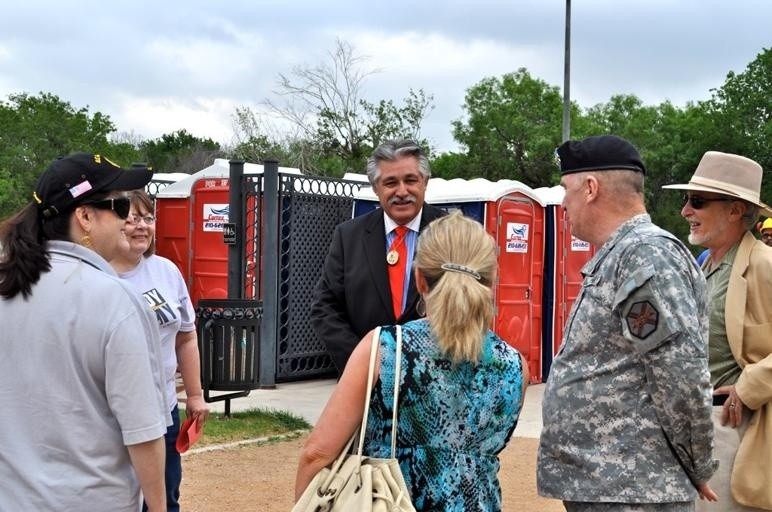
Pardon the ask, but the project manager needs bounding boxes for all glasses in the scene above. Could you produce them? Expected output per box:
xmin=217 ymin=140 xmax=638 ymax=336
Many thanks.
xmin=678 ymin=193 xmax=734 ymax=209
xmin=125 ymin=214 xmax=158 ymax=227
xmin=79 ymin=195 xmax=130 ymax=219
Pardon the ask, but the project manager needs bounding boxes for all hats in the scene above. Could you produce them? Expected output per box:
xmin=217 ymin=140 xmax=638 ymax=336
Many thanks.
xmin=662 ymin=151 xmax=765 ymax=207
xmin=557 ymin=136 xmax=645 ymax=174
xmin=761 ymin=218 xmax=772 ymax=231
xmin=30 ymin=152 xmax=154 ymax=220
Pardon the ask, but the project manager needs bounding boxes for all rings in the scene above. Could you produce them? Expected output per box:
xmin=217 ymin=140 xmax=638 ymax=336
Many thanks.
xmin=729 ymin=402 xmax=735 ymax=410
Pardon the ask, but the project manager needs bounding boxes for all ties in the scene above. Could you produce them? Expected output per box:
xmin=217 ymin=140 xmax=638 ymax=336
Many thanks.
xmin=388 ymin=225 xmax=406 ymax=320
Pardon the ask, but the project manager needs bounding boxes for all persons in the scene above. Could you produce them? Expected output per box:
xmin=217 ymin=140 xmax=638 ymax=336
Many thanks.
xmin=294 ymin=211 xmax=529 ymax=512
xmin=538 ymin=135 xmax=717 ymax=512
xmin=107 ymin=190 xmax=208 ymax=512
xmin=663 ymin=150 xmax=772 ymax=512
xmin=0 ymin=154 xmax=168 ymax=512
xmin=760 ymin=218 xmax=772 ymax=247
xmin=312 ymin=138 xmax=449 ymax=379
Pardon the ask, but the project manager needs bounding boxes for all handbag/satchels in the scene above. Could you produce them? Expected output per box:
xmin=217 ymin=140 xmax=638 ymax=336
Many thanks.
xmin=290 ymin=324 xmax=415 ymax=512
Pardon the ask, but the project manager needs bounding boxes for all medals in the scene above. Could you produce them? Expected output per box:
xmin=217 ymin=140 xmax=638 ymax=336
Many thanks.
xmin=385 ymin=218 xmax=417 ymax=266
